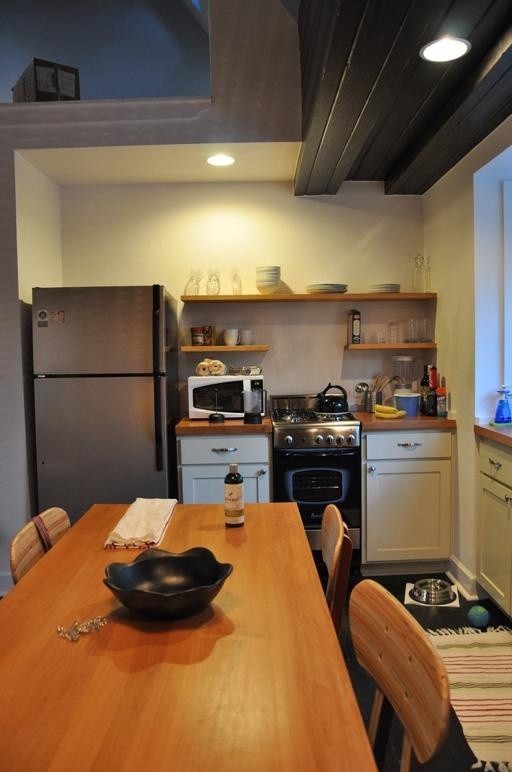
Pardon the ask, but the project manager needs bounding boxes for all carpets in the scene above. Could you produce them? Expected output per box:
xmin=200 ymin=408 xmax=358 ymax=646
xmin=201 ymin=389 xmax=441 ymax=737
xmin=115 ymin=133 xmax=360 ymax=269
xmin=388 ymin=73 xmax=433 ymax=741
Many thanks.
xmin=424 ymin=626 xmax=512 ymax=771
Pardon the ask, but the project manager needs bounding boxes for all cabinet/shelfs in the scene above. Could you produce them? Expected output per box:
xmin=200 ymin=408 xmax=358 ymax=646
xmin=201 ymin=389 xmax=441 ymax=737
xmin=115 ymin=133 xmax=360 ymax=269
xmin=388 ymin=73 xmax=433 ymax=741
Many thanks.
xmin=176 ymin=432 xmax=273 ymax=504
xmin=352 ymin=429 xmax=457 ymax=577
xmin=178 ymin=290 xmax=437 ymax=353
xmin=475 ymin=437 xmax=512 ymax=619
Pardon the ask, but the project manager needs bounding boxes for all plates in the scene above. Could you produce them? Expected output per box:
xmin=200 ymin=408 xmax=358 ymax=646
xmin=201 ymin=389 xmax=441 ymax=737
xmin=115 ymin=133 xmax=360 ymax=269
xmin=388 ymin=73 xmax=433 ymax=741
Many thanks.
xmin=368 ymin=283 xmax=401 ymax=292
xmin=306 ymin=283 xmax=348 ymax=293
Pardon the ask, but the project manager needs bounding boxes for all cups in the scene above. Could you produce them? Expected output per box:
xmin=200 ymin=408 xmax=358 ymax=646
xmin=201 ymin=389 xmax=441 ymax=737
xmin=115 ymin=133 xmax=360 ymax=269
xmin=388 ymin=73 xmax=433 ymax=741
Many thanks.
xmin=373 ymin=331 xmax=386 ymax=343
xmin=224 ymin=328 xmax=241 ymax=345
xmin=361 ymin=331 xmax=373 ymax=343
xmin=387 ymin=321 xmax=399 ymax=344
xmin=240 ymin=329 xmax=254 ymax=346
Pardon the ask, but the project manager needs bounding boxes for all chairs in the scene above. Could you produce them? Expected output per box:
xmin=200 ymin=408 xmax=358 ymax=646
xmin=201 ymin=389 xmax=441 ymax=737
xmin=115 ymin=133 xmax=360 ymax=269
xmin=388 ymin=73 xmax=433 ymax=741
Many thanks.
xmin=349 ymin=578 xmax=450 ymax=771
xmin=319 ymin=504 xmax=351 ymax=642
xmin=11 ymin=507 xmax=71 ymax=587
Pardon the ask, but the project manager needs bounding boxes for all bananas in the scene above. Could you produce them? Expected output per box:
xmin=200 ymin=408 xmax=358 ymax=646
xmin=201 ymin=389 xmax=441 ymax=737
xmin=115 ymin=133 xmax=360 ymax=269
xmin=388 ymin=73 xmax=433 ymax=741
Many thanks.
xmin=375 ymin=404 xmax=408 ymax=419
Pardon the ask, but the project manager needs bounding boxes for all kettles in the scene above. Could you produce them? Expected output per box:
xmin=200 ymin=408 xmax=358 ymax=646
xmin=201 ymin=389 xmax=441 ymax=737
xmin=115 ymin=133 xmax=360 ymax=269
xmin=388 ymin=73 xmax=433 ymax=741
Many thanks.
xmin=313 ymin=382 xmax=349 ymax=412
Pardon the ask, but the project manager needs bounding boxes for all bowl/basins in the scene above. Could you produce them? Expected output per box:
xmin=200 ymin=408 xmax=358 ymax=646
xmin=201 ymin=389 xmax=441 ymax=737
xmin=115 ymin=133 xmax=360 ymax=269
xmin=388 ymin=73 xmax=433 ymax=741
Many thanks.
xmin=255 ymin=265 xmax=282 ymax=295
xmin=101 ymin=546 xmax=234 ymax=619
xmin=409 ymin=578 xmax=457 ymax=605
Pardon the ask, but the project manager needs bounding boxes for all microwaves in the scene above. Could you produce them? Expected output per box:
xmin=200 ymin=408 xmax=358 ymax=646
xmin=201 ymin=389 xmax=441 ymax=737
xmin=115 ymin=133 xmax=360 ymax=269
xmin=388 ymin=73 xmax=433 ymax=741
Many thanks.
xmin=187 ymin=375 xmax=268 ymax=420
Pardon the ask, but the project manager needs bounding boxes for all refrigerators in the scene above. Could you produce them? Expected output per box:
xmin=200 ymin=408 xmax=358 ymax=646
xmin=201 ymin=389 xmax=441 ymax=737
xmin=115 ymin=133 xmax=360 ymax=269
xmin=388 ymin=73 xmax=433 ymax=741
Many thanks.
xmin=31 ymin=284 xmax=181 ymax=514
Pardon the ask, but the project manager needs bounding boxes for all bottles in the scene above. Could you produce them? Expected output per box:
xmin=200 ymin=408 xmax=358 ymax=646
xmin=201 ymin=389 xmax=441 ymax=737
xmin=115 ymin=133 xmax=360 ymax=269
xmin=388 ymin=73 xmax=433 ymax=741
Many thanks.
xmin=414 ymin=255 xmax=427 ymax=293
xmin=421 ymin=364 xmax=429 ymax=412
xmin=348 ymin=305 xmax=362 ymax=344
xmin=190 ymin=326 xmax=203 ymax=346
xmin=223 ymin=464 xmax=245 ymax=529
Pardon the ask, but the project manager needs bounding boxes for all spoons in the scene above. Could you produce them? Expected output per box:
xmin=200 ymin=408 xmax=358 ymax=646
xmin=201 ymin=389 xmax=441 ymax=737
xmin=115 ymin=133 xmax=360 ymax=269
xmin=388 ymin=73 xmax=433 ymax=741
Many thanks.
xmin=355 ymin=382 xmax=370 ymax=392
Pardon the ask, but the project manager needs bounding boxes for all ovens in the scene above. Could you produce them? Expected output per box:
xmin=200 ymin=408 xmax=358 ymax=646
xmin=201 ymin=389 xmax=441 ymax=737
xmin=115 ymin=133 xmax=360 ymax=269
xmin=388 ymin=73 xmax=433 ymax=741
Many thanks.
xmin=272 ymin=446 xmax=362 ymax=530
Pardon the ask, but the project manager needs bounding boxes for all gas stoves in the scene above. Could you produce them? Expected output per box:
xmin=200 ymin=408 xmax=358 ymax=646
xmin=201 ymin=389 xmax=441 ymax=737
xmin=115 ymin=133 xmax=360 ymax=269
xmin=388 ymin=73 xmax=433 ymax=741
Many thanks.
xmin=268 ymin=410 xmax=361 ymax=449
